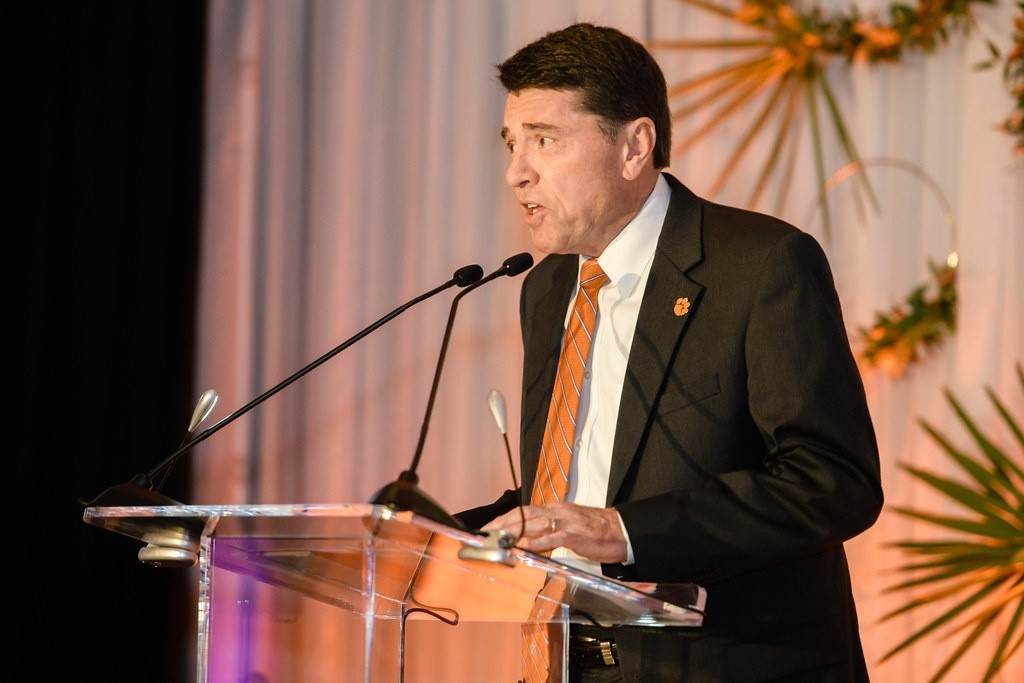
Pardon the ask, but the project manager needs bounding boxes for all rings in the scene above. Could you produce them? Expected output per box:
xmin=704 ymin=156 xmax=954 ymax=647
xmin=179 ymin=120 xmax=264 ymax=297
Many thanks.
xmin=550 ymin=517 xmax=557 ymax=532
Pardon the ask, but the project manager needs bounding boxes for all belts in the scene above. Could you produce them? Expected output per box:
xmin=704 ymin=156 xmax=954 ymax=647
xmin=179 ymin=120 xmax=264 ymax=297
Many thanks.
xmin=570 ymin=635 xmax=621 ymax=667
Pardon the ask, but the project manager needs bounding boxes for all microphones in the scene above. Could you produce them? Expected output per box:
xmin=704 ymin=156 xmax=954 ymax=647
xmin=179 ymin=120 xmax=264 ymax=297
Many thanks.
xmin=367 ymin=253 xmax=534 ymax=535
xmin=90 ymin=264 xmax=484 ymax=507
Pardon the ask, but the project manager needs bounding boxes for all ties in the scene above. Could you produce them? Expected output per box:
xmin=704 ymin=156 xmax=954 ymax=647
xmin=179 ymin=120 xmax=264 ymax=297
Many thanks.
xmin=521 ymin=573 xmax=570 ymax=683
xmin=529 ymin=258 xmax=607 ymax=559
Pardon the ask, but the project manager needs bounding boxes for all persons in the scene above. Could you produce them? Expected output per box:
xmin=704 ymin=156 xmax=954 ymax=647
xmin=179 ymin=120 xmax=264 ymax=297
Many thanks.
xmin=447 ymin=22 xmax=886 ymax=683
